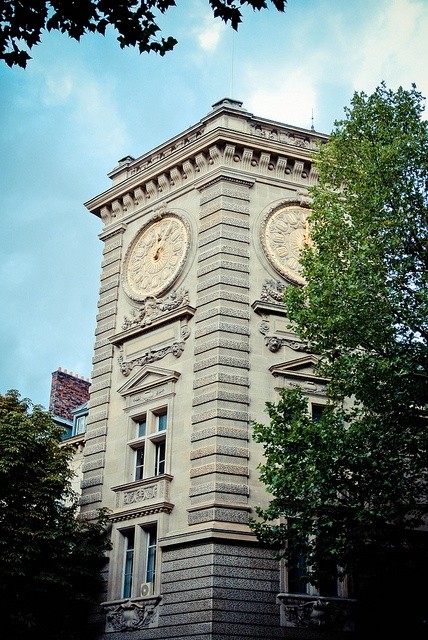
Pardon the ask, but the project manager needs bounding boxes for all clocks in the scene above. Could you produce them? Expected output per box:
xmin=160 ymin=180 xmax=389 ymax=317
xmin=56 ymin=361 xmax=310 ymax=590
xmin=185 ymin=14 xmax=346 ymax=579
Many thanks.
xmin=120 ymin=209 xmax=198 ymax=307
xmin=252 ymin=196 xmax=350 ymax=289
